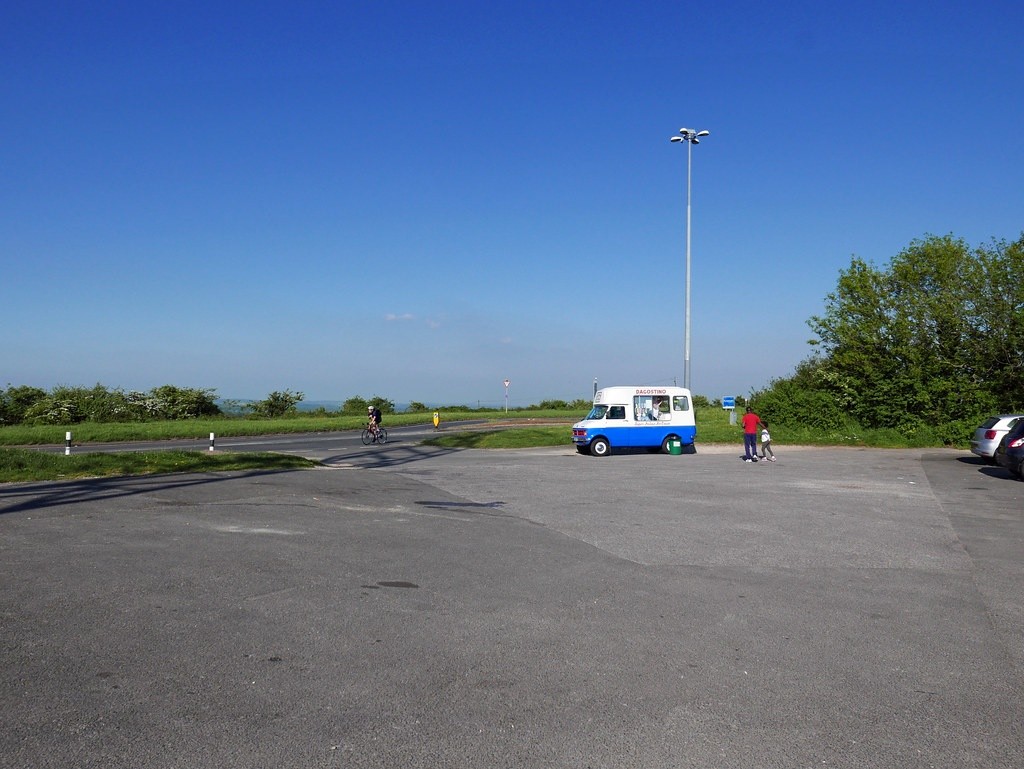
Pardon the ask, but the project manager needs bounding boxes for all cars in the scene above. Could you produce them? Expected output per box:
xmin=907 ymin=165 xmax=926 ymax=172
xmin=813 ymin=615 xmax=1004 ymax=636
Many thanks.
xmin=968 ymin=416 xmax=1024 ymax=479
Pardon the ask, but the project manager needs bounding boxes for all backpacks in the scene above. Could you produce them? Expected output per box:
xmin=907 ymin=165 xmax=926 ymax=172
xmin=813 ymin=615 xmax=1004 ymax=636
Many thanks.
xmin=375 ymin=408 xmax=382 ymax=418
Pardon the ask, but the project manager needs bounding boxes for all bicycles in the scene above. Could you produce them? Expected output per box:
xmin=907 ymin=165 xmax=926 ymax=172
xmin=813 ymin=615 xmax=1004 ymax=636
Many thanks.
xmin=361 ymin=423 xmax=387 ymax=445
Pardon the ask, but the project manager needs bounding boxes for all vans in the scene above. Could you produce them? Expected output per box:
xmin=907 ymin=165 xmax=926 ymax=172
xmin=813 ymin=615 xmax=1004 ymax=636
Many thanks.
xmin=570 ymin=386 xmax=696 ymax=457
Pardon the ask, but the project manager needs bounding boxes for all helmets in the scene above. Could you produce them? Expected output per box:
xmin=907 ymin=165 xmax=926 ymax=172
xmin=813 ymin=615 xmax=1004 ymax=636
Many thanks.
xmin=368 ymin=406 xmax=374 ymax=409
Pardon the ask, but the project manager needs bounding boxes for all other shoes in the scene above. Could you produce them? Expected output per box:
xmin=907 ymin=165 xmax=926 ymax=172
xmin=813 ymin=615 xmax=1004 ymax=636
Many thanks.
xmin=375 ymin=430 xmax=380 ymax=435
xmin=372 ymin=438 xmax=376 ymax=443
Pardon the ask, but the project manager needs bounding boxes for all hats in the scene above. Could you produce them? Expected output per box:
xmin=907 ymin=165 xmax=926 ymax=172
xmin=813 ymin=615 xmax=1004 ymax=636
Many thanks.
xmin=746 ymin=406 xmax=754 ymax=411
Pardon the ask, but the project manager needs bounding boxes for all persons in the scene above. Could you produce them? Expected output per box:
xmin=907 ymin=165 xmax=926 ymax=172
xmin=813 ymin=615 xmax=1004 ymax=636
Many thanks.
xmin=760 ymin=421 xmax=776 ymax=462
xmin=367 ymin=406 xmax=381 ymax=434
xmin=742 ymin=407 xmax=768 ymax=462
xmin=653 ymin=400 xmax=662 ymax=420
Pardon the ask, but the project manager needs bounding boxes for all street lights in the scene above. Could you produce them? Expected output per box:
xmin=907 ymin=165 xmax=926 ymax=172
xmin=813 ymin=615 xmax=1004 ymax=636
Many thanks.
xmin=671 ymin=128 xmax=709 ymax=411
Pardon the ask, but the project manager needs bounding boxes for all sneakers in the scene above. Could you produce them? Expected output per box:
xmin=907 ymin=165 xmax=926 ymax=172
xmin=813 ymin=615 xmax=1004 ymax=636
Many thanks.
xmin=762 ymin=457 xmax=767 ymax=461
xmin=745 ymin=459 xmax=752 ymax=463
xmin=770 ymin=456 xmax=776 ymax=461
xmin=753 ymin=455 xmax=758 ymax=461
